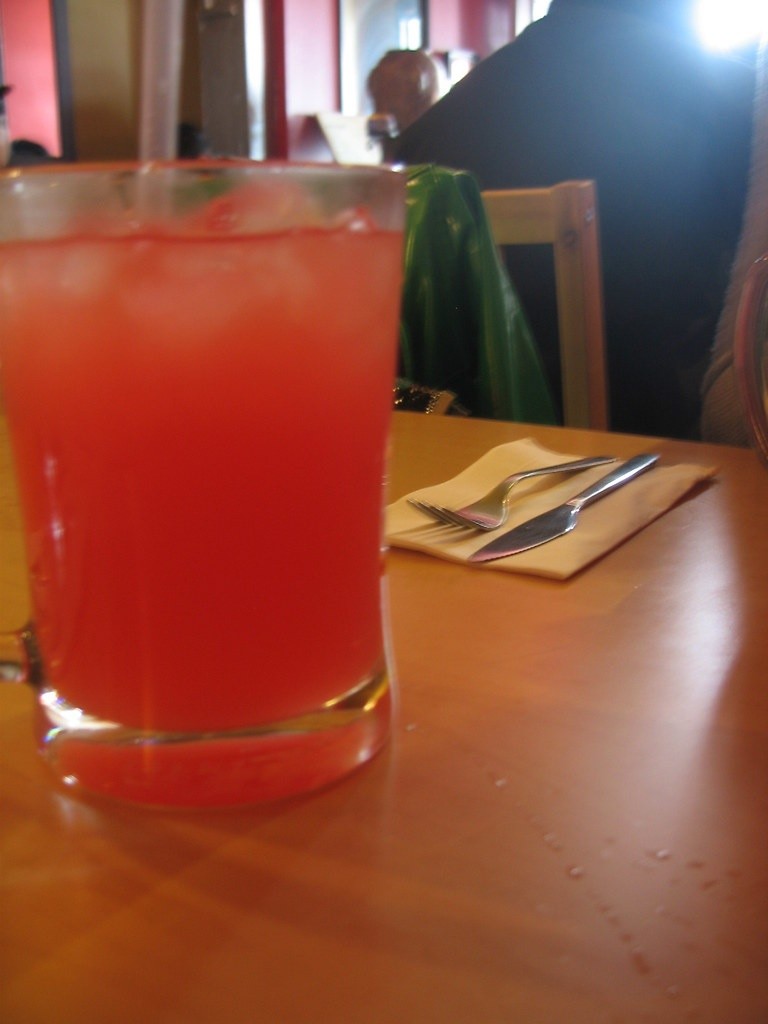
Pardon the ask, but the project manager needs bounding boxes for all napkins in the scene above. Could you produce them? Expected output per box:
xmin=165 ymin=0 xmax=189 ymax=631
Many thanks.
xmin=385 ymin=434 xmax=716 ymax=586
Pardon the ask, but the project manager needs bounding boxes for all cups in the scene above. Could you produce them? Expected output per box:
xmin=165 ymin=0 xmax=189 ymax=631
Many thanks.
xmin=0 ymin=163 xmax=409 ymax=811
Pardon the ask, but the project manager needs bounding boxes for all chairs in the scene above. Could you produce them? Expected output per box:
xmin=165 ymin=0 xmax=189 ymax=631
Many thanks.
xmin=392 ymin=179 xmax=611 ymax=434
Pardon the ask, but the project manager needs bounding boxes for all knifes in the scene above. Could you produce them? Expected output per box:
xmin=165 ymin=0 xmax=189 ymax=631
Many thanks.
xmin=467 ymin=452 xmax=662 ymax=564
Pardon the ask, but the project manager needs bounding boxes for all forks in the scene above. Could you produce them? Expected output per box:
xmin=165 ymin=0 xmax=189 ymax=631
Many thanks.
xmin=408 ymin=456 xmax=614 ymax=532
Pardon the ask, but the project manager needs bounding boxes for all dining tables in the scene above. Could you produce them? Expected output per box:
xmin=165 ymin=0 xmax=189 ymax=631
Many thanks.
xmin=0 ymin=407 xmax=768 ymax=1024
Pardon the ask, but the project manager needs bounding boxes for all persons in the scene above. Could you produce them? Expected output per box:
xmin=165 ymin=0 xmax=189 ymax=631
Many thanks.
xmin=386 ymin=0 xmax=768 ymax=445
